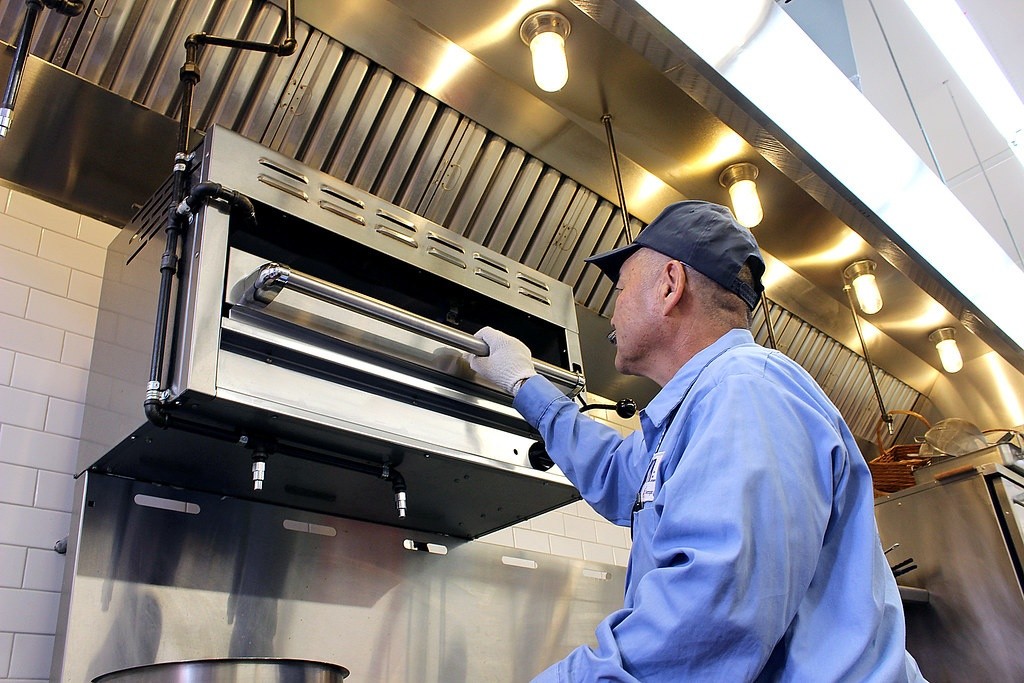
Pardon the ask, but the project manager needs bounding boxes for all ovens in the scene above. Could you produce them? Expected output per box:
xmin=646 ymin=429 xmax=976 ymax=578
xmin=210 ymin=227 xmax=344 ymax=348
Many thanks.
xmin=72 ymin=121 xmax=590 ymax=542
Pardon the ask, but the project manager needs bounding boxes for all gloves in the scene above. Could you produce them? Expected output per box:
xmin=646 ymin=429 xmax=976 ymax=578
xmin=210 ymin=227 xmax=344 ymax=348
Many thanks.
xmin=467 ymin=326 xmax=538 ymax=398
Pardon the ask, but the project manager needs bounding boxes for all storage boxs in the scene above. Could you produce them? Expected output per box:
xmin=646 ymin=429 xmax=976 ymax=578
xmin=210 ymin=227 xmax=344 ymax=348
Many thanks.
xmin=911 ymin=441 xmax=1015 ymax=485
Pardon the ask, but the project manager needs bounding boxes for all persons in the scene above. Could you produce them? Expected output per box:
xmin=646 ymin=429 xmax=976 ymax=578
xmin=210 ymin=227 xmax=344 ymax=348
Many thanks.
xmin=469 ymin=199 xmax=930 ymax=683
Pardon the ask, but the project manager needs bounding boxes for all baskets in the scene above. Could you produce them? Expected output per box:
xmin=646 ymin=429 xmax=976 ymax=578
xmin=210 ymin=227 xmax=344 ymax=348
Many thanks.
xmin=869 ymin=411 xmax=932 ymax=493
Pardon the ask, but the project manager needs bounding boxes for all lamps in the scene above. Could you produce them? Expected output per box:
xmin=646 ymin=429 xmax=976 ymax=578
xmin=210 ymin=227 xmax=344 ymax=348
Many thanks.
xmin=519 ymin=10 xmax=572 ymax=94
xmin=928 ymin=326 xmax=964 ymax=374
xmin=718 ymin=162 xmax=766 ymax=229
xmin=844 ymin=259 xmax=883 ymax=316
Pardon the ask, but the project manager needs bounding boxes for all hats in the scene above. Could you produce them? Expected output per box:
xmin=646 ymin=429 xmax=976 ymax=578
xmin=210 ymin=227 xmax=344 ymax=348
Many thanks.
xmin=583 ymin=200 xmax=765 ymax=312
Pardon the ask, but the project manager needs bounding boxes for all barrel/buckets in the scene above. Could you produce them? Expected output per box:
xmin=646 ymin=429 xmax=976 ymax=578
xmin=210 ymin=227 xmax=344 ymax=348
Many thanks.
xmin=90 ymin=656 xmax=350 ymax=682
